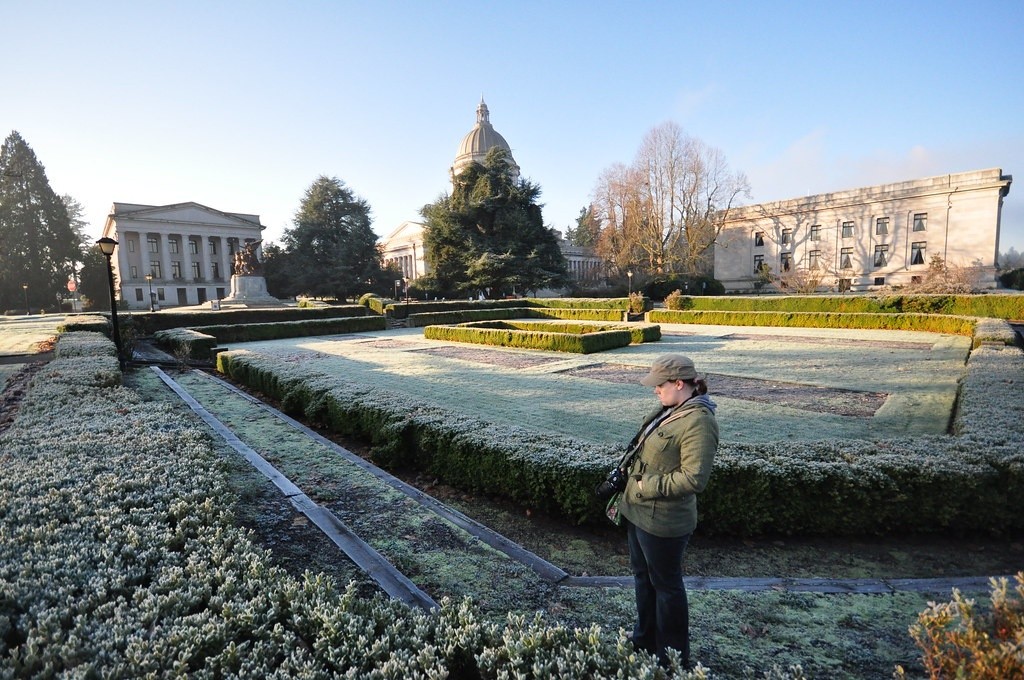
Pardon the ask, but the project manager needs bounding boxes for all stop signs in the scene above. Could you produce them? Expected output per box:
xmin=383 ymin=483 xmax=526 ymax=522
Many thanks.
xmin=67 ymin=281 xmax=77 ymax=292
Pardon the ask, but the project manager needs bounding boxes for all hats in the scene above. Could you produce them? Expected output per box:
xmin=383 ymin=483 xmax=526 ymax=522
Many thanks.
xmin=639 ymin=354 xmax=697 ymax=387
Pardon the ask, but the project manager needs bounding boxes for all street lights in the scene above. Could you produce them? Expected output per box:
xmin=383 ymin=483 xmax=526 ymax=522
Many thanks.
xmin=402 ymin=276 xmax=410 ymax=304
xmin=95 ymin=237 xmax=125 ymax=373
xmin=22 ymin=283 xmax=30 ymax=315
xmin=627 ymin=270 xmax=634 ymax=298
xmin=145 ymin=274 xmax=155 ymax=312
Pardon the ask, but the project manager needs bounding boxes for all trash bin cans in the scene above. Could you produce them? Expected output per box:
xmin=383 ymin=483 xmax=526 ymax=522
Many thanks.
xmin=211 ymin=300 xmax=221 ymax=311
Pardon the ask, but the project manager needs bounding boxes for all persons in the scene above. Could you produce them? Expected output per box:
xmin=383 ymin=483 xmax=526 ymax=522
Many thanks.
xmin=234 ymin=242 xmax=254 ymax=276
xmin=608 ymin=354 xmax=719 ymax=668
